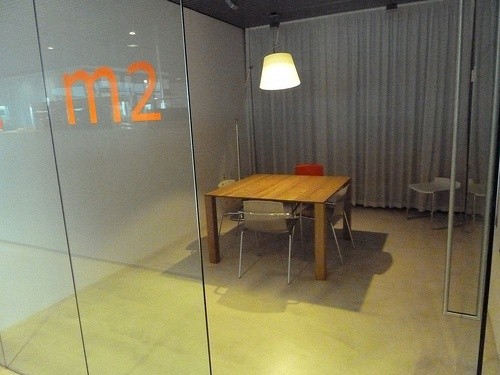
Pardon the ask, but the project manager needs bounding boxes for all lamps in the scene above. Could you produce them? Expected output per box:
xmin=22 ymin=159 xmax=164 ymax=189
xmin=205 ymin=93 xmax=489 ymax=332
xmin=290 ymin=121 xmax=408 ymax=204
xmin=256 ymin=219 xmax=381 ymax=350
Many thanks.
xmin=258 ymin=14 xmax=302 ymax=93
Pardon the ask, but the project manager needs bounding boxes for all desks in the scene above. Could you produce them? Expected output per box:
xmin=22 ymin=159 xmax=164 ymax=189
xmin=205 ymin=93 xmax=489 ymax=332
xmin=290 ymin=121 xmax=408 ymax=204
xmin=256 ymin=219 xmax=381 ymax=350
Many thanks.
xmin=202 ymin=170 xmax=354 ymax=281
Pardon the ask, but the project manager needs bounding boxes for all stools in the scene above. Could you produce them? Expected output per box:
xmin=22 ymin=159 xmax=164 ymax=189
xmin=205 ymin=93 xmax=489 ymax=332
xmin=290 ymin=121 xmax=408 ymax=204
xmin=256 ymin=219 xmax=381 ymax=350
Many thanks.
xmin=467 ymin=179 xmax=487 ymax=231
xmin=403 ymin=177 xmax=462 ymax=228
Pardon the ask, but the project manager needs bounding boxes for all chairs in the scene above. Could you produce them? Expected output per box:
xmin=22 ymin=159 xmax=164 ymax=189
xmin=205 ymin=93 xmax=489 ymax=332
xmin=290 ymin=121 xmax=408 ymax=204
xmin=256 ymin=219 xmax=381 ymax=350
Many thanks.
xmin=296 ymin=180 xmax=354 ymax=264
xmin=236 ymin=199 xmax=299 ymax=285
xmin=216 ymin=179 xmax=264 ymax=249
xmin=293 ymin=162 xmax=325 ymax=176
